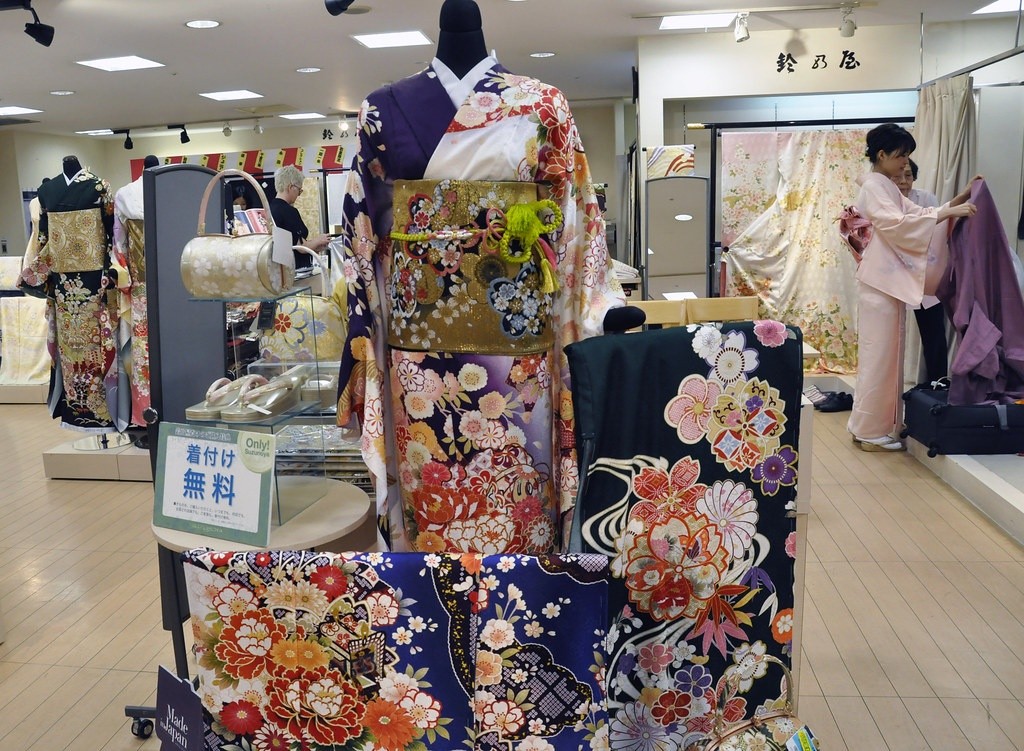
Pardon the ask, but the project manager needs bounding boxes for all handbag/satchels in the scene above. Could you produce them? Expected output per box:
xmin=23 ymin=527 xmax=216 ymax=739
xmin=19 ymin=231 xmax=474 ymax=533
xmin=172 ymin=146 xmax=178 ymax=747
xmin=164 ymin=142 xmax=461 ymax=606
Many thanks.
xmin=180 ymin=169 xmax=295 ymax=298
xmin=259 ymin=245 xmax=347 ymax=362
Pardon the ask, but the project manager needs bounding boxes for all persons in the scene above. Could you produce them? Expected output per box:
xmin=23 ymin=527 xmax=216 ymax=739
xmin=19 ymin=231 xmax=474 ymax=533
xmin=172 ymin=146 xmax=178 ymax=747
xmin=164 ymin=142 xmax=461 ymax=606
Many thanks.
xmin=224 ymin=186 xmax=253 ymax=236
xmin=16 ymin=155 xmax=159 ymax=435
xmin=337 ymin=0 xmax=626 ymax=555
xmin=846 ymin=124 xmax=984 ymax=453
xmin=270 ymin=167 xmax=332 ymax=268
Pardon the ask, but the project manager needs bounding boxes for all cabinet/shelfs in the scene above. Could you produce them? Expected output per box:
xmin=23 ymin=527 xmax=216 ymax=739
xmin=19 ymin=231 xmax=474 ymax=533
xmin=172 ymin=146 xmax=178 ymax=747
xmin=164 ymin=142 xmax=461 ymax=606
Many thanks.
xmin=182 ymin=282 xmax=327 ymax=527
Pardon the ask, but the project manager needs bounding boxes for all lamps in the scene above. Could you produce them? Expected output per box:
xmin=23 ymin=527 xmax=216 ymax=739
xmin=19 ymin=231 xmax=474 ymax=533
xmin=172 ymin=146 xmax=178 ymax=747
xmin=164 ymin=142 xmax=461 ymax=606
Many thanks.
xmin=838 ymin=4 xmax=856 ymax=37
xmin=23 ymin=0 xmax=54 ymax=46
xmin=735 ymin=12 xmax=752 ymax=42
xmin=325 ymin=0 xmax=355 ymax=16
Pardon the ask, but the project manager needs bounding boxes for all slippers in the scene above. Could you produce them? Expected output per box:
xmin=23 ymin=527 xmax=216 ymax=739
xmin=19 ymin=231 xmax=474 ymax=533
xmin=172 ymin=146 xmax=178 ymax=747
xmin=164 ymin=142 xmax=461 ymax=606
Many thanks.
xmin=185 ymin=372 xmax=261 ymax=421
xmin=299 ymin=371 xmax=338 ymax=408
xmin=850 ymin=434 xmax=907 ymax=451
xmin=220 ymin=373 xmax=301 ymax=422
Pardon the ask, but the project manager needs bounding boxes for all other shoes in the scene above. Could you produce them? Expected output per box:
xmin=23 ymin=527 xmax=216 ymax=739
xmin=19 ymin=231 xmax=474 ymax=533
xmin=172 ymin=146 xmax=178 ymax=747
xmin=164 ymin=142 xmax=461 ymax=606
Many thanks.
xmin=813 ymin=391 xmax=846 ymax=408
xmin=820 ymin=394 xmax=854 ymax=412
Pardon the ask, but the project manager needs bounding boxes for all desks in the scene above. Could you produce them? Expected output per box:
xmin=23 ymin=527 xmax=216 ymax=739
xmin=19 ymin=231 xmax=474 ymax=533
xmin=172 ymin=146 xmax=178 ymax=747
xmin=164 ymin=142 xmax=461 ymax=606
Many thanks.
xmin=151 ymin=475 xmax=370 ymax=687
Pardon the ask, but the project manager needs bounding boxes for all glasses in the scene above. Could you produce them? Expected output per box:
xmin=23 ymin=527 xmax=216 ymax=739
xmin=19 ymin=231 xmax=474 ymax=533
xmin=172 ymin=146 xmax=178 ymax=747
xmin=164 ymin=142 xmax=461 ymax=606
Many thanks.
xmin=290 ymin=185 xmax=303 ymax=196
